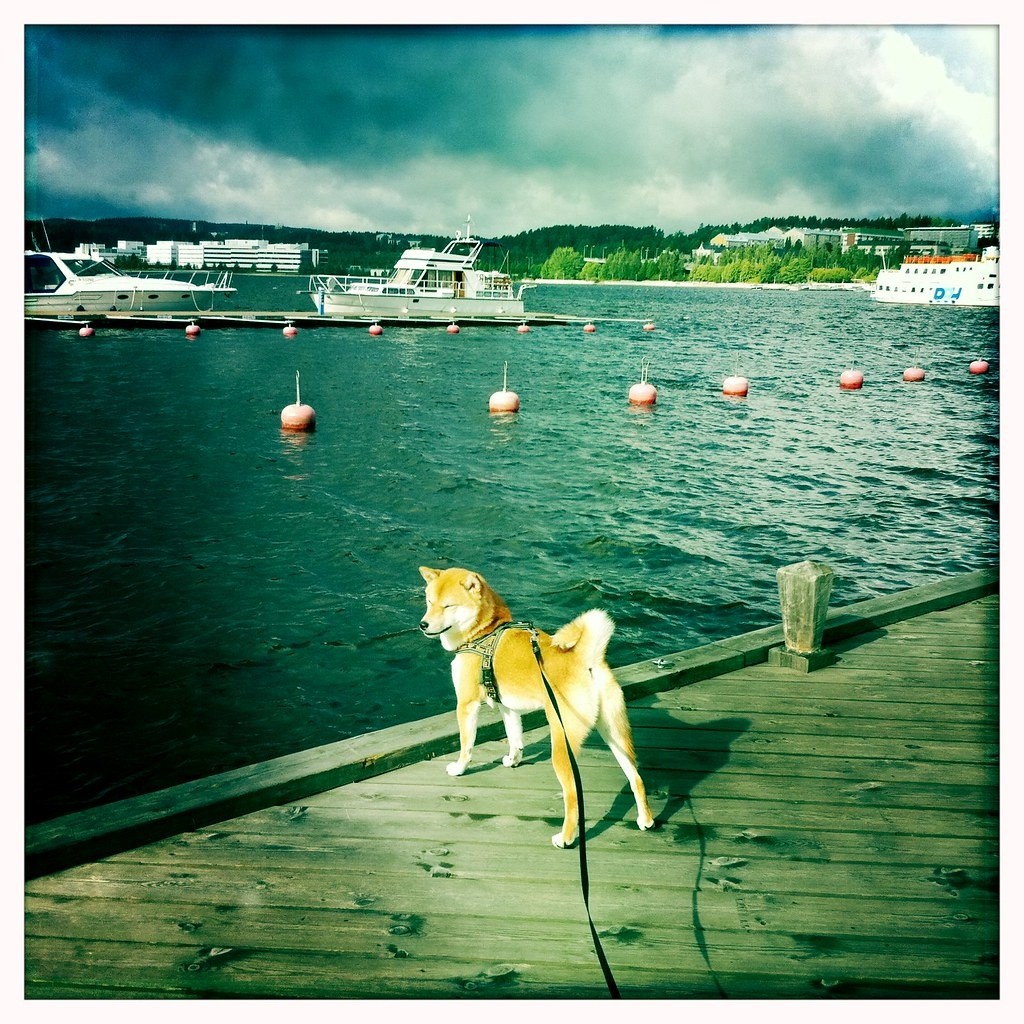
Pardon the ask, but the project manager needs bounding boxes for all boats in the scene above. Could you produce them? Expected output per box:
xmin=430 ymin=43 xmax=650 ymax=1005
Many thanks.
xmin=870 ymin=245 xmax=1000 ymax=306
xmin=24 ymin=250 xmax=238 ymax=311
xmin=296 ymin=214 xmax=537 ymax=312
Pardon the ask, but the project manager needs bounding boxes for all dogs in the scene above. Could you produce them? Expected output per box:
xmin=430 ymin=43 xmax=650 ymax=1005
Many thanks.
xmin=418 ymin=566 xmax=656 ymax=849
